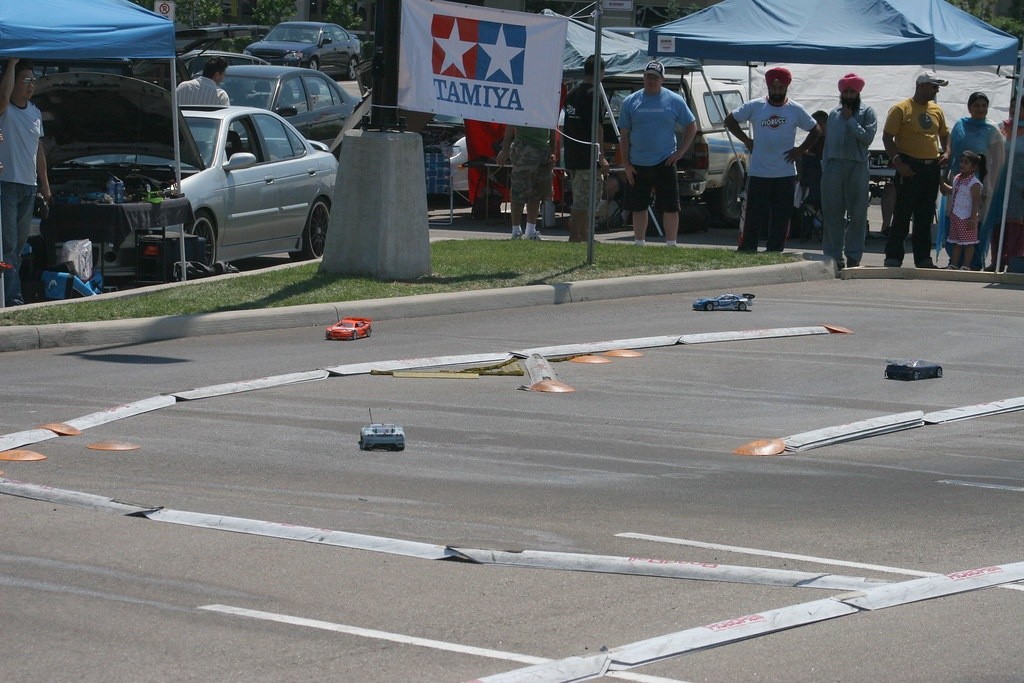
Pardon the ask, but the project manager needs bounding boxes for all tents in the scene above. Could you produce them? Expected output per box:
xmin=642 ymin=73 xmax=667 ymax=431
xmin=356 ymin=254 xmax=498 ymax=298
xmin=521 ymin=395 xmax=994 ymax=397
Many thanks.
xmin=0 ymin=0 xmax=188 ymax=280
xmin=647 ymin=0 xmax=1023 ymax=272
xmin=538 ymin=8 xmax=746 ymax=179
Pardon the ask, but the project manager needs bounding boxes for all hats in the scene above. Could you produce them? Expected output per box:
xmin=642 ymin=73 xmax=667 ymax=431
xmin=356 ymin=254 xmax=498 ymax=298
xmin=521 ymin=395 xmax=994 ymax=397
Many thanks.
xmin=765 ymin=67 xmax=792 ymax=86
xmin=644 ymin=60 xmax=665 ymax=78
xmin=916 ymin=74 xmax=949 ymax=87
xmin=838 ymin=73 xmax=865 ymax=92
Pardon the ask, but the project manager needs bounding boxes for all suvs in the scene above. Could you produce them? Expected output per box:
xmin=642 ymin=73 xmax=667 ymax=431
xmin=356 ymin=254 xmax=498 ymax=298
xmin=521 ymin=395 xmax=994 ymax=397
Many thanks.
xmin=563 ymin=74 xmax=760 ymax=230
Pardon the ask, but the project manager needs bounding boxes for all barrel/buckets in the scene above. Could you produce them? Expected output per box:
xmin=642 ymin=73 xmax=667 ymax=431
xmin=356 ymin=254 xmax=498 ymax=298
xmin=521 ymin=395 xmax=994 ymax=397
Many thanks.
xmin=175 ymin=233 xmax=198 ymax=282
xmin=143 ymin=232 xmax=180 ymax=284
xmin=198 ymin=237 xmax=207 ymax=278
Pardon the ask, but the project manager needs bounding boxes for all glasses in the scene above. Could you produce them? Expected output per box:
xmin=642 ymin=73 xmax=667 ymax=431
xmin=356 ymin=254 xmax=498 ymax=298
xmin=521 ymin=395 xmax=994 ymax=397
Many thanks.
xmin=927 ymin=83 xmax=940 ymax=90
xmin=17 ymin=78 xmax=37 ymax=85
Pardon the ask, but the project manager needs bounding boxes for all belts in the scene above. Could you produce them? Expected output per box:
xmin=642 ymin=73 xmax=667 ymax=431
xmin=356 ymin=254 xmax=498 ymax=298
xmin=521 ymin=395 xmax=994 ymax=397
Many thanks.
xmin=898 ymin=154 xmax=939 ymax=165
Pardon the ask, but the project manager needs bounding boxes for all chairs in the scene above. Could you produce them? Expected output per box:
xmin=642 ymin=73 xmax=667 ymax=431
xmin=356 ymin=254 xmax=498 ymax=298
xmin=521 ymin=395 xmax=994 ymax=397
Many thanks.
xmin=251 ymin=82 xmax=271 ymax=109
xmin=225 ymin=129 xmax=244 ymax=157
xmin=295 ymin=82 xmax=321 ymax=113
xmin=797 ymin=153 xmax=824 ymax=245
xmin=422 ymin=144 xmax=454 ymax=225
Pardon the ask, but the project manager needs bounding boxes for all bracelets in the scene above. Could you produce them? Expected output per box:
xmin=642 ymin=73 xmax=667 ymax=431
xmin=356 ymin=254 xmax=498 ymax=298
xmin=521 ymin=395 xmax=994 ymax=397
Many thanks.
xmin=889 ymin=155 xmax=900 ymax=164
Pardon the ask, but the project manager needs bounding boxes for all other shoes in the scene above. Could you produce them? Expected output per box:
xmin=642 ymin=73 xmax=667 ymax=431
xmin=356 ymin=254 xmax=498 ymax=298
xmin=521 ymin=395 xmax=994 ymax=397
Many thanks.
xmin=970 ymin=264 xmax=981 ymax=271
xmin=837 ymin=263 xmax=845 ymax=274
xmin=916 ymin=264 xmax=938 ymax=268
xmin=984 ymin=263 xmax=1004 ymax=272
xmin=525 ymin=231 xmax=542 ymax=241
xmin=511 ymin=232 xmax=525 ymax=240
xmin=946 ymin=265 xmax=959 ymax=269
xmin=959 ymin=266 xmax=971 ymax=270
xmin=803 ymin=231 xmax=812 ymax=240
xmin=802 ymin=196 xmax=816 ymax=204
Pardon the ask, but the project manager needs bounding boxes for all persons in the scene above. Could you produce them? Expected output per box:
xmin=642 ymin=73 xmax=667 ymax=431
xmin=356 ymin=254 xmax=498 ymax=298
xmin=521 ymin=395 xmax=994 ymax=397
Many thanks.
xmin=984 ymin=92 xmax=1024 ymax=274
xmin=176 ymin=55 xmax=233 ymax=132
xmin=0 ymin=57 xmax=52 ymax=306
xmin=883 ymin=70 xmax=951 ymax=268
xmin=805 ymin=110 xmax=830 ymax=158
xmin=618 ymin=60 xmax=699 ymax=245
xmin=945 ymin=150 xmax=988 ymax=271
xmin=936 ymin=91 xmax=1005 ymax=271
xmin=561 ymin=55 xmax=609 ymax=243
xmin=725 ymin=68 xmax=822 ymax=255
xmin=494 ymin=123 xmax=555 ymax=241
xmin=822 ymin=72 xmax=876 ymax=269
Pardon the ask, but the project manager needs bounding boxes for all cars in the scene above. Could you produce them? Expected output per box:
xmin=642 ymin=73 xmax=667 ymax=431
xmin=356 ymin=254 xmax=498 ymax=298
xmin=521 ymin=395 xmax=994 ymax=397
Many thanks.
xmin=243 ymin=21 xmax=364 ymax=82
xmin=360 ymin=421 xmax=404 ymax=453
xmin=135 ymin=25 xmax=364 ymax=151
xmin=692 ymin=291 xmax=756 ymax=311
xmin=420 ymin=114 xmax=566 ymax=207
xmin=23 ymin=71 xmax=341 ymax=286
xmin=325 ymin=316 xmax=372 ymax=341
xmin=885 ymin=357 xmax=944 ymax=381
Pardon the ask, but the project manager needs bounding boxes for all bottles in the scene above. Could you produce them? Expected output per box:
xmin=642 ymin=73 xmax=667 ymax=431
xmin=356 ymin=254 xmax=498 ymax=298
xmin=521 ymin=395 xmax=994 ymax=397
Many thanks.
xmin=106 ymin=176 xmax=115 ymax=202
xmin=115 ymin=180 xmax=124 ymax=204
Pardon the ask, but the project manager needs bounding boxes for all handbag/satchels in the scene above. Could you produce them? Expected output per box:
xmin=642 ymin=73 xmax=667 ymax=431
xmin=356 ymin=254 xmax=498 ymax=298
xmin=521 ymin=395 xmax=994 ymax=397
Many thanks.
xmin=32 ymin=196 xmax=50 ymax=219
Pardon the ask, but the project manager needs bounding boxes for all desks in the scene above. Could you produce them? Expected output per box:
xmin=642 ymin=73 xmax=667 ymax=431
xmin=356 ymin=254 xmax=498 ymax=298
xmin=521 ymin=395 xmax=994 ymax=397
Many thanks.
xmin=463 ymin=160 xmax=686 ymax=231
xmin=40 ymin=198 xmax=195 ymax=293
xmin=869 ymin=168 xmax=896 ymax=176
